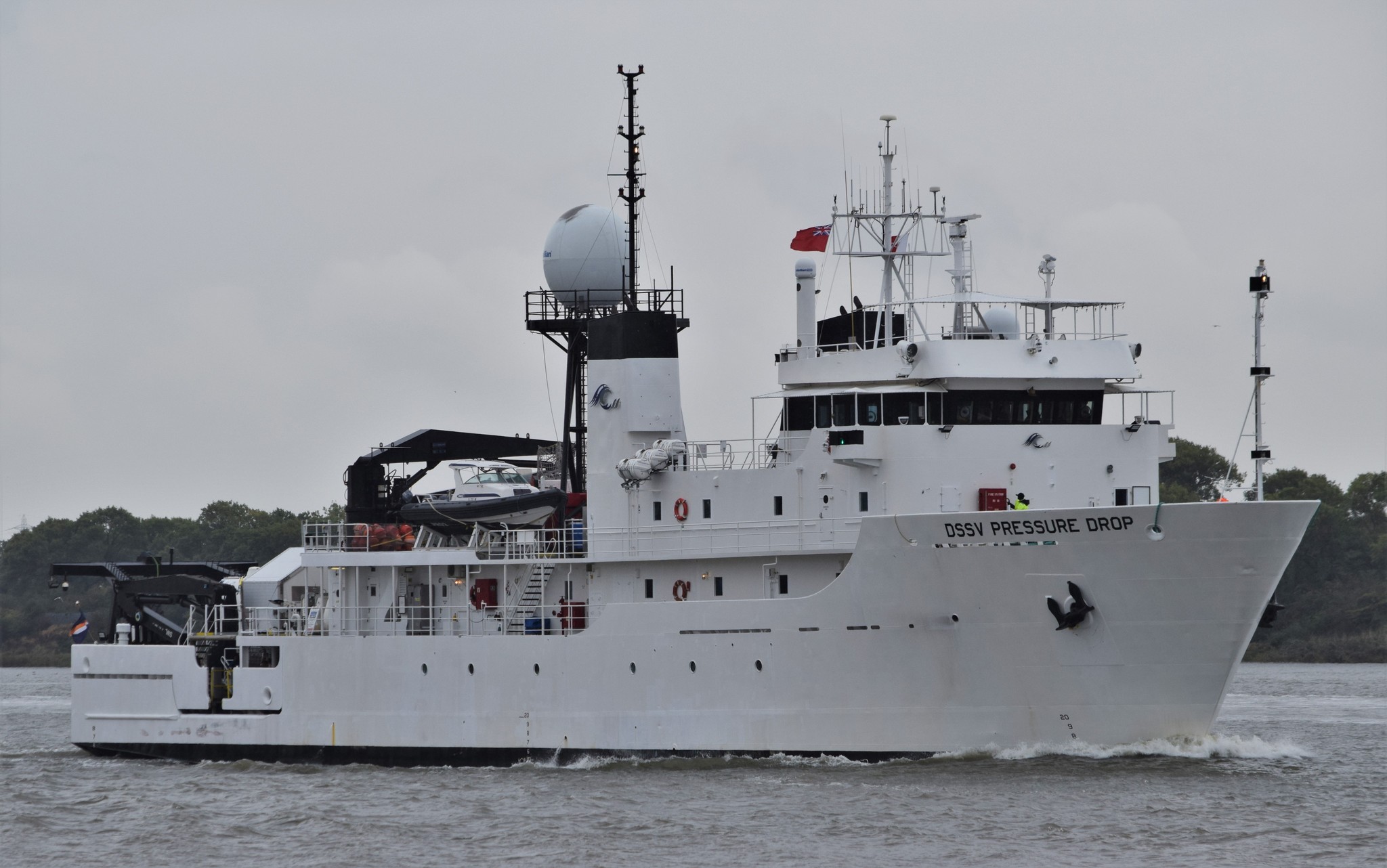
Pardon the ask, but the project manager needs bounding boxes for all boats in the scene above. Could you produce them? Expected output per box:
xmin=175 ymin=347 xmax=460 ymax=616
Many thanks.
xmin=45 ymin=60 xmax=1324 ymax=755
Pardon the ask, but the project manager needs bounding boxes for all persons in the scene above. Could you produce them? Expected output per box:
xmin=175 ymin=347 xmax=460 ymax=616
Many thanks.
xmin=1008 ymin=493 xmax=1029 ymax=510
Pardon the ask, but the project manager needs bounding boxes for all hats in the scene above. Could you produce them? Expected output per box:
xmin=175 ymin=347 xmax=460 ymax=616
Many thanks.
xmin=1016 ymin=493 xmax=1025 ymax=497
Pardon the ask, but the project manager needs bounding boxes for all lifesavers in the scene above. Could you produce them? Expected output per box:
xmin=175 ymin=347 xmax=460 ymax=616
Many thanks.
xmin=674 ymin=498 xmax=688 ymax=521
xmin=673 ymin=581 xmax=688 ymax=602
xmin=470 ymin=585 xmax=476 ymax=606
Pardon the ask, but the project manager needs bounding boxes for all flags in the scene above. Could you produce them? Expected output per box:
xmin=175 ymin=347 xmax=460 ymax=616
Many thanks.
xmin=69 ymin=607 xmax=90 ymax=644
xmin=883 ymin=236 xmax=898 ymax=260
xmin=790 ymin=224 xmax=833 ymax=252
